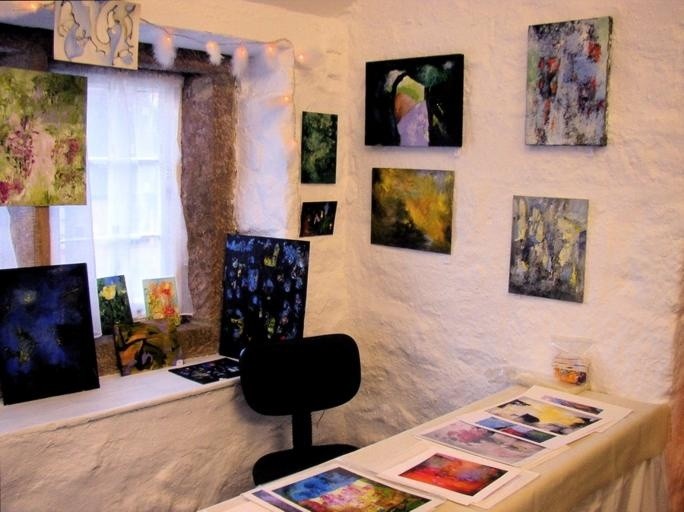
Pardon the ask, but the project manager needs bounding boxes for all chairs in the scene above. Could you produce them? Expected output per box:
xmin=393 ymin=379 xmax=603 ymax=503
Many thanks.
xmin=238 ymin=334 xmax=363 ymax=490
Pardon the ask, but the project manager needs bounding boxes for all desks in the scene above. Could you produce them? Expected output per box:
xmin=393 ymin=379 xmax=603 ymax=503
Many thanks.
xmin=190 ymin=379 xmax=669 ymax=512
